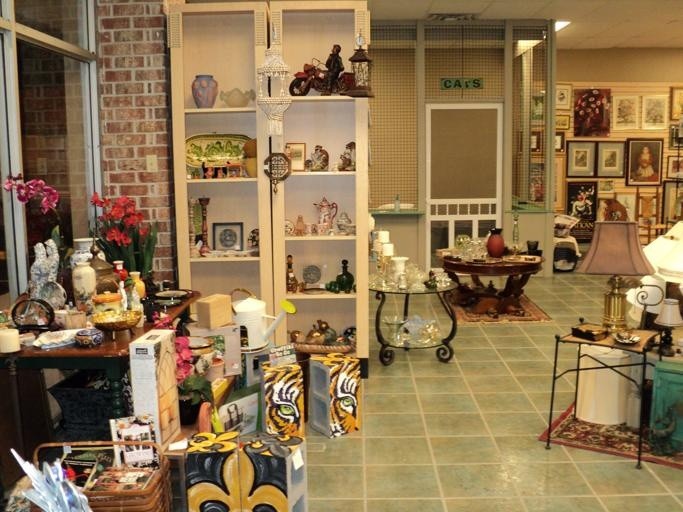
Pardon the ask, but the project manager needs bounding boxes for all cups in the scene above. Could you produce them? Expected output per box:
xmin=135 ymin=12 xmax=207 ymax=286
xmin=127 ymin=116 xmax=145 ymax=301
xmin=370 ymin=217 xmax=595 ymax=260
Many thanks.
xmin=384 ymin=320 xmax=408 ymax=343
xmin=527 ymin=240 xmax=540 ymax=253
xmin=451 ymin=240 xmax=486 ymax=262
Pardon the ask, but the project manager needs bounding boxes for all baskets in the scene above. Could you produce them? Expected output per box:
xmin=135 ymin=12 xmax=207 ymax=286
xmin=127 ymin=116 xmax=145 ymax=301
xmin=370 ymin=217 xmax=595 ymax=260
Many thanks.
xmin=32 ymin=441 xmax=175 ymax=512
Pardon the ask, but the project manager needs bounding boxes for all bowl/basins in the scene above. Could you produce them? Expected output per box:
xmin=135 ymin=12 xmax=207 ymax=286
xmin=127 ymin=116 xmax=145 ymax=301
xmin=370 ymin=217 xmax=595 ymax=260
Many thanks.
xmin=90 ymin=309 xmax=143 ymax=331
xmin=40 ymin=282 xmax=68 ymax=311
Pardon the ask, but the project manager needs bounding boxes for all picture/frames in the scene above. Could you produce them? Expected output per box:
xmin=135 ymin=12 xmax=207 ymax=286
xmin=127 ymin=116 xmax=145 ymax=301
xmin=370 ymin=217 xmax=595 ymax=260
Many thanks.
xmin=283 ymin=141 xmax=307 ymax=173
xmin=524 ymin=82 xmax=683 ymax=243
xmin=211 ymin=222 xmax=244 ymax=252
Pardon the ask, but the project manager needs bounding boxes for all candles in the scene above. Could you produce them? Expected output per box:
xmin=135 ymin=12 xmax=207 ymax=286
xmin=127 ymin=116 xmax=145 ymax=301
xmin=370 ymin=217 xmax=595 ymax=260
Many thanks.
xmin=1 ymin=329 xmax=21 ymax=354
xmin=376 ymin=229 xmax=390 ymax=243
xmin=380 ymin=243 xmax=394 ymax=256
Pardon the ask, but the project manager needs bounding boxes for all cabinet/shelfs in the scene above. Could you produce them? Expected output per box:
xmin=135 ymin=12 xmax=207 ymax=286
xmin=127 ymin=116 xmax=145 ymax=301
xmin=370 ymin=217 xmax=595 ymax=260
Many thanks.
xmin=268 ymin=0 xmax=377 ymax=379
xmin=165 ymin=1 xmax=273 ymax=348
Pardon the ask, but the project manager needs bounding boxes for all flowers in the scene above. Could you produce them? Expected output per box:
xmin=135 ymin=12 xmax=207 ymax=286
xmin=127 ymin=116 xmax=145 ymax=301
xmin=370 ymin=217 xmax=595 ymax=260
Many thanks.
xmin=0 ymin=171 xmax=76 ymax=273
xmin=81 ymin=188 xmax=162 ymax=284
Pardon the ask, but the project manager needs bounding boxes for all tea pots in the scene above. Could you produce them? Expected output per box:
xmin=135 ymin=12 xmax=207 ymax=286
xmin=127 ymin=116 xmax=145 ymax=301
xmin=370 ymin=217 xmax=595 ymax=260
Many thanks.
xmin=313 ymin=197 xmax=338 ymax=232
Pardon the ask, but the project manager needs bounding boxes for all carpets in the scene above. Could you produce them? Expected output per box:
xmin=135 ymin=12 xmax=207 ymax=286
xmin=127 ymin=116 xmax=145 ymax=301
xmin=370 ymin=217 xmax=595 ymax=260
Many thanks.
xmin=535 ymin=400 xmax=683 ymax=473
xmin=437 ymin=281 xmax=554 ymax=327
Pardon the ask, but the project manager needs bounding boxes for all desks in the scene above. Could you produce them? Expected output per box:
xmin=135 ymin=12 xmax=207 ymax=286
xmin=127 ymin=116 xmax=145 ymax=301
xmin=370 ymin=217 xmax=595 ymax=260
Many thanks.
xmin=0 ymin=287 xmax=204 ymax=491
xmin=541 ymin=325 xmax=662 ymax=472
xmin=365 ymin=263 xmax=463 ymax=367
xmin=437 ymin=245 xmax=547 ymax=319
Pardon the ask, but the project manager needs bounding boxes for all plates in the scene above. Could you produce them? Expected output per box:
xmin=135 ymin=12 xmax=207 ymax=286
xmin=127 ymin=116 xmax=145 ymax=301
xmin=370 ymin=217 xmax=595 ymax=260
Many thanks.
xmin=220 ymin=228 xmax=236 ymax=248
xmin=377 ymin=203 xmax=414 ymax=209
xmin=284 ymin=219 xmax=296 ymax=236
xmin=153 ymin=299 xmax=181 ymax=308
xmin=153 ymin=291 xmax=189 ymax=298
xmin=246 ymin=228 xmax=259 ymax=257
xmin=185 ymin=132 xmax=251 ymax=169
xmin=616 ymin=333 xmax=640 ymax=346
xmin=301 ymin=264 xmax=322 ymax=285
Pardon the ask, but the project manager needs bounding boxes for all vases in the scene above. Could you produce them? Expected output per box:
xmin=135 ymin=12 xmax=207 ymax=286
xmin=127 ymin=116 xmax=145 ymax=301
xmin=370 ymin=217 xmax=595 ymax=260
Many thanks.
xmin=128 ymin=271 xmax=146 ymax=302
xmin=54 ymin=273 xmax=74 ymax=301
xmin=113 ymin=260 xmax=127 ymax=284
xmin=69 ymin=237 xmax=106 ymax=271
xmin=70 ymin=260 xmax=102 ymax=314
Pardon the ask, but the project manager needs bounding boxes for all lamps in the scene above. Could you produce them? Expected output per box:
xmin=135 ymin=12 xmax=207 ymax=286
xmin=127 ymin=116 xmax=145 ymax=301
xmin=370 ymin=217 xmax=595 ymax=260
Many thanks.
xmin=347 ymin=30 xmax=375 ymax=99
xmin=648 ymin=297 xmax=683 ymax=358
xmin=574 ymin=218 xmax=655 ymax=337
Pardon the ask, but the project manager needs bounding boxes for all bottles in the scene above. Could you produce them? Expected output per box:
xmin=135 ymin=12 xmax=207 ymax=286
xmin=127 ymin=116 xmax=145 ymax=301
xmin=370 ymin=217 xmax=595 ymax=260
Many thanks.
xmin=488 ymin=227 xmax=505 ymax=256
xmin=295 ymin=215 xmax=306 ymax=235
xmin=398 ymin=272 xmax=407 ymax=290
xmin=424 ymin=271 xmax=439 ymax=288
xmin=113 ymin=261 xmax=128 ymax=279
xmin=130 ymin=270 xmax=145 ymax=298
xmin=70 ymin=236 xmax=109 ymax=316
xmin=335 ymin=259 xmax=355 ymax=292
xmin=394 ymin=193 xmax=400 ymax=214
xmin=285 ymin=255 xmax=298 ymax=293
xmin=310 ymin=145 xmax=329 ymax=171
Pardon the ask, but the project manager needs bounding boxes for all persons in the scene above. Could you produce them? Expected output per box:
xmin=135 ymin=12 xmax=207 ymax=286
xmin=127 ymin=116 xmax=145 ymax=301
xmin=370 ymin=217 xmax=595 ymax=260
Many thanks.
xmin=633 ymin=145 xmax=657 ymax=181
xmin=320 ymin=45 xmax=344 ymax=96
